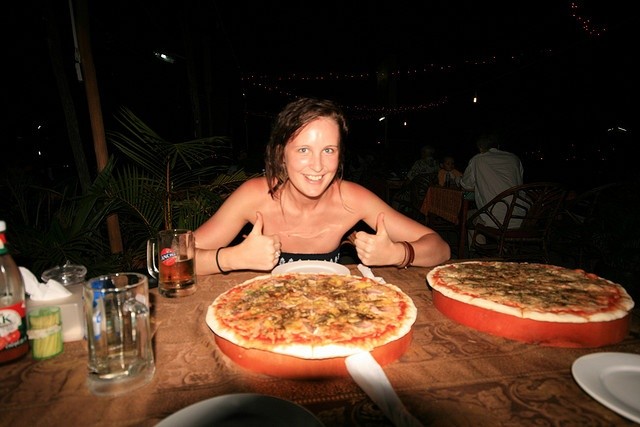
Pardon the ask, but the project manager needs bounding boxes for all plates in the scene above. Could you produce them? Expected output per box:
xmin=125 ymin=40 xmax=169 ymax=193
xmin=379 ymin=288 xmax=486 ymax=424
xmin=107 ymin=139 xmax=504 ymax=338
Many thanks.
xmin=571 ymin=352 xmax=639 ymax=424
xmin=271 ymin=260 xmax=350 ymax=277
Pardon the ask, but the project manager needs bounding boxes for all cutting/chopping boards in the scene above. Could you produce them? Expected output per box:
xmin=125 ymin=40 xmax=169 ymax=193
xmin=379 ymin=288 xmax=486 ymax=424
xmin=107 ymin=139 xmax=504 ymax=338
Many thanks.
xmin=433 ymin=280 xmax=629 ymax=346
xmin=214 ymin=322 xmax=415 ymax=381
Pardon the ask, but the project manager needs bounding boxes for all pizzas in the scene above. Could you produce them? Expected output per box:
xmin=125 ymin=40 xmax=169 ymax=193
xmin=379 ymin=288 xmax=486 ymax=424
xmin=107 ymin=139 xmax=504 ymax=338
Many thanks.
xmin=425 ymin=261 xmax=636 ymax=324
xmin=205 ymin=273 xmax=417 ymax=359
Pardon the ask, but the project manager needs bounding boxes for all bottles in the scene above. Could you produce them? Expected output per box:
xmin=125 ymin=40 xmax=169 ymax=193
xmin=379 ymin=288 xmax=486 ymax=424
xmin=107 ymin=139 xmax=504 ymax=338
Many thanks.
xmin=0 ymin=221 xmax=27 ymax=362
xmin=26 ymin=307 xmax=64 ymax=360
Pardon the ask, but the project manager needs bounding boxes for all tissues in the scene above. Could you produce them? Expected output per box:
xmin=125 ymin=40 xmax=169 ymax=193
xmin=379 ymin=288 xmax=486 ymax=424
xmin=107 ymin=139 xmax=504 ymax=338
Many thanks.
xmin=19 ymin=267 xmax=85 ymax=342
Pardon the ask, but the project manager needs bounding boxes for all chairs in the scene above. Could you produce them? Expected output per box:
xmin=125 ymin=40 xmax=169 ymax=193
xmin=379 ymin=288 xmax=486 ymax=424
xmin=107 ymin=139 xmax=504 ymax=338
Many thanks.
xmin=465 ymin=182 xmax=563 ymax=257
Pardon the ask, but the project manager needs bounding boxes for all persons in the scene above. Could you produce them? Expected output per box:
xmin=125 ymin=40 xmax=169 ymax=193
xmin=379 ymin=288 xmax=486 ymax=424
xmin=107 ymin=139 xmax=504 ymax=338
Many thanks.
xmin=438 ymin=157 xmax=465 ymax=186
xmin=169 ymin=97 xmax=451 ymax=277
xmin=408 ymin=145 xmax=440 ymax=196
xmin=459 ymin=137 xmax=532 ymax=228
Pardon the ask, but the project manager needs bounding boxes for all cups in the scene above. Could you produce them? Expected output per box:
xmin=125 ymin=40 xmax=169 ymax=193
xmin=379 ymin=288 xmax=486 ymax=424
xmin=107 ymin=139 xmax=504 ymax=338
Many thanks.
xmin=146 ymin=230 xmax=196 ymax=298
xmin=42 ymin=263 xmax=87 ymax=342
xmin=86 ymin=274 xmax=156 ymax=396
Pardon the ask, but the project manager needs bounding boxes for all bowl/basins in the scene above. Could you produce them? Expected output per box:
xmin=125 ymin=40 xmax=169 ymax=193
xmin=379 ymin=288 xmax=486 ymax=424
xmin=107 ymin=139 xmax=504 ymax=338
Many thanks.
xmin=156 ymin=393 xmax=324 ymax=426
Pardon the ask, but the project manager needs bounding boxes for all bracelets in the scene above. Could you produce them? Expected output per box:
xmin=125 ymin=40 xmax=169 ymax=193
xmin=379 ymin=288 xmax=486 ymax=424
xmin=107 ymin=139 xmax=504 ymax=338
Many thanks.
xmin=396 ymin=240 xmax=414 ymax=269
xmin=216 ymin=247 xmax=230 ymax=274
